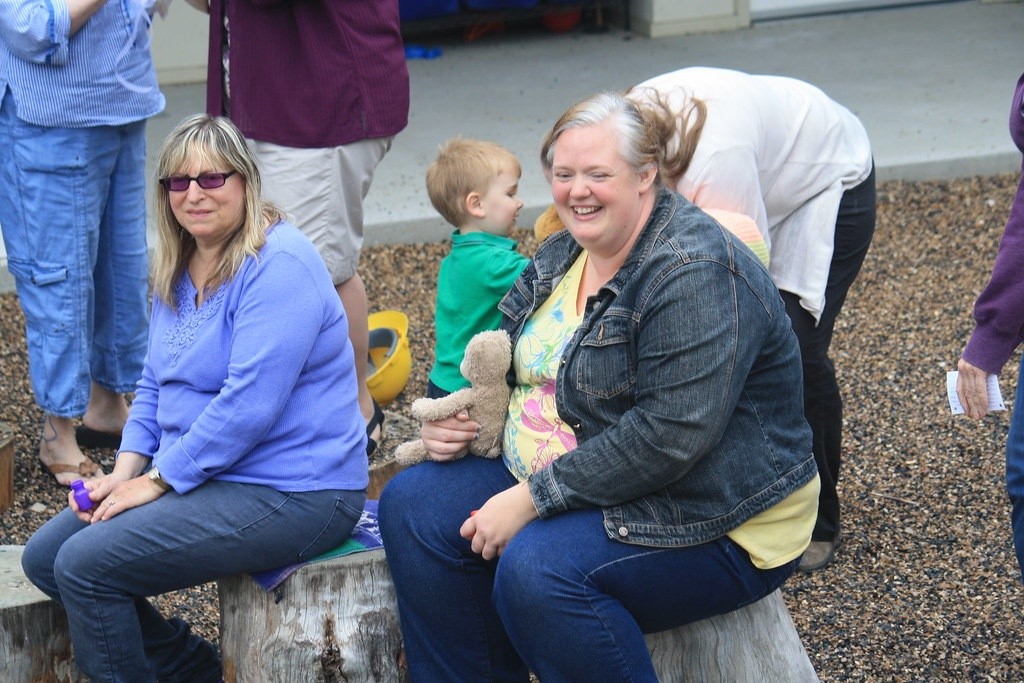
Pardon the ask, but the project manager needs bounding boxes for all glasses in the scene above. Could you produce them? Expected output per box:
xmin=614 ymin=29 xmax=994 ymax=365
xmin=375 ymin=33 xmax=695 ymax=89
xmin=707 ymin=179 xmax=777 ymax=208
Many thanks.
xmin=159 ymin=169 xmax=236 ymax=191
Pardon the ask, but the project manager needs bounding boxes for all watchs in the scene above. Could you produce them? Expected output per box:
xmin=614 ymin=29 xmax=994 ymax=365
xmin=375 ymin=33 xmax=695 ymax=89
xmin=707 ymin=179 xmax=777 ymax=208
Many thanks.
xmin=148 ymin=468 xmax=170 ymax=491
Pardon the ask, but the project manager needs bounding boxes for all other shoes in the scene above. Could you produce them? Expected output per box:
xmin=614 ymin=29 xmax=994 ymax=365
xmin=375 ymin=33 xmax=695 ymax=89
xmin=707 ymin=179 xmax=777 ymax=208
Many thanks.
xmin=798 ymin=529 xmax=843 ymax=570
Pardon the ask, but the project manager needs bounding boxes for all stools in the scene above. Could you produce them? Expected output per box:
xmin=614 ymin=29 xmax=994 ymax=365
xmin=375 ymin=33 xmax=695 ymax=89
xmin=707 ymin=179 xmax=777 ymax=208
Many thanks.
xmin=0 ymin=545 xmax=93 ymax=683
xmin=644 ymin=585 xmax=820 ymax=683
xmin=218 ymin=498 xmax=409 ymax=683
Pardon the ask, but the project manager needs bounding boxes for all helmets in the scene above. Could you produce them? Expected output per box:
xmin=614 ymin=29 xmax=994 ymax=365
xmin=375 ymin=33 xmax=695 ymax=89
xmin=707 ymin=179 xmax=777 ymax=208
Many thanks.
xmin=365 ymin=309 xmax=412 ymax=404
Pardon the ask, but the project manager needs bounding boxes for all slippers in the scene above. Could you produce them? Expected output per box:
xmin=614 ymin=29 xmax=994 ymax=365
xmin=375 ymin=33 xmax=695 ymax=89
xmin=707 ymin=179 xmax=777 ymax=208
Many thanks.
xmin=39 ymin=458 xmax=99 ymax=489
xmin=75 ymin=424 xmax=122 ymax=449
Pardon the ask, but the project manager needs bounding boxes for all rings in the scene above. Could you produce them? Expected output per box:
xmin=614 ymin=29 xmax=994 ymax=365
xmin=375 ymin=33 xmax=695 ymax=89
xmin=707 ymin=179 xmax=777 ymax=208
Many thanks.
xmin=109 ymin=500 xmax=115 ymax=505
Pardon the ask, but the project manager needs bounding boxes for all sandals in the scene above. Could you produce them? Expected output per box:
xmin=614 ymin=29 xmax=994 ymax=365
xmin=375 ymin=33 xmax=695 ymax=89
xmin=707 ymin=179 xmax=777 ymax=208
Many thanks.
xmin=367 ymin=399 xmax=386 ymax=458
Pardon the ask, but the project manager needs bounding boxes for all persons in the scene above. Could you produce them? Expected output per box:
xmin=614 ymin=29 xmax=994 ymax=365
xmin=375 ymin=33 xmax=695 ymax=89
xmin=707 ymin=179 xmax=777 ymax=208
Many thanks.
xmin=187 ymin=0 xmax=411 ymax=461
xmin=0 ymin=0 xmax=172 ymax=487
xmin=955 ymin=75 xmax=1024 ymax=573
xmin=378 ymin=85 xmax=821 ymax=682
xmin=429 ymin=137 xmax=530 ymax=406
xmin=625 ymin=66 xmax=877 ymax=570
xmin=20 ymin=111 xmax=371 ymax=682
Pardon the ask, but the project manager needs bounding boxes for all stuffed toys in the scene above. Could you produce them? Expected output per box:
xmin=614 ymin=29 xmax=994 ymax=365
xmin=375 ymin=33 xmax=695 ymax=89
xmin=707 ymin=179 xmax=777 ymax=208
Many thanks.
xmin=393 ymin=329 xmax=514 ymax=466
